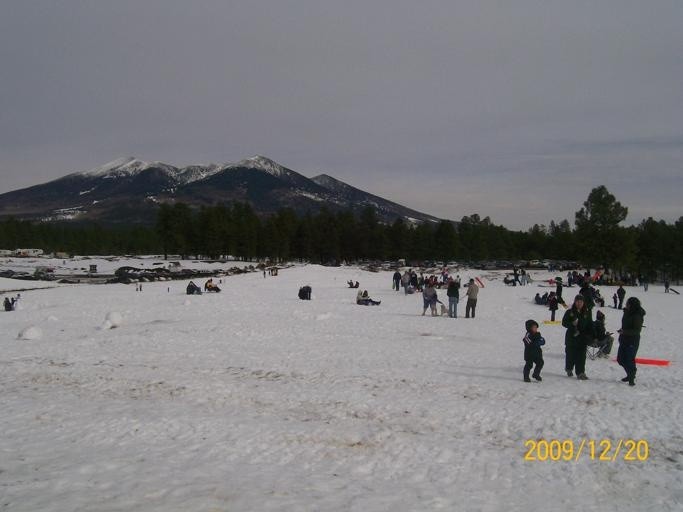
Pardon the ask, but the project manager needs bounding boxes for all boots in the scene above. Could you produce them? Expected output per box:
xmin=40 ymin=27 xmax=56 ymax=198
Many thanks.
xmin=622 ymin=368 xmax=637 ymax=386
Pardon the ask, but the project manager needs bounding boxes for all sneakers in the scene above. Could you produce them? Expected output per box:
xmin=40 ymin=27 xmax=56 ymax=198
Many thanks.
xmin=565 ymin=367 xmax=573 ymax=376
xmin=577 ymin=373 xmax=587 ymax=380
xmin=532 ymin=372 xmax=541 ymax=381
xmin=524 ymin=376 xmax=530 ymax=382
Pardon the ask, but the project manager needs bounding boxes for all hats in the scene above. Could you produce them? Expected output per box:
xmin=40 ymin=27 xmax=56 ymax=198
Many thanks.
xmin=575 ymin=294 xmax=585 ymax=301
xmin=597 ymin=310 xmax=605 ymax=320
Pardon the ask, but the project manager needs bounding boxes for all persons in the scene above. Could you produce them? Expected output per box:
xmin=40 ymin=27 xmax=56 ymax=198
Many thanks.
xmin=263 ymin=267 xmax=278 ymax=277
xmin=562 ymin=293 xmax=614 ymax=380
xmin=392 ymin=263 xmax=479 ymax=318
xmin=504 ymin=262 xmax=669 ymax=322
xmin=522 ymin=320 xmax=546 ymax=382
xmin=205 ymin=279 xmax=222 ymax=293
xmin=616 ymin=296 xmax=646 ymax=387
xmin=348 ymin=280 xmax=381 ymax=305
xmin=186 ymin=280 xmax=203 ymax=295
